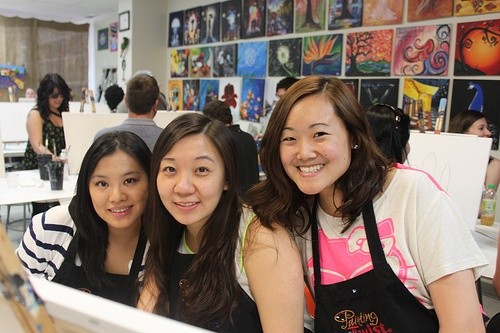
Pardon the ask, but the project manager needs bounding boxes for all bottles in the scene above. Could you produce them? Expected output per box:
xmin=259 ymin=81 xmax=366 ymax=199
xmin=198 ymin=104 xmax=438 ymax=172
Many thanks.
xmin=481 ymin=183 xmax=496 ymax=226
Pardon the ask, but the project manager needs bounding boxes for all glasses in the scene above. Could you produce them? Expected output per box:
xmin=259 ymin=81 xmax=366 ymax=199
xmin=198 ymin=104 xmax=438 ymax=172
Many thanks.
xmin=374 ymin=101 xmax=402 ymax=131
xmin=50 ymin=93 xmax=60 ymax=99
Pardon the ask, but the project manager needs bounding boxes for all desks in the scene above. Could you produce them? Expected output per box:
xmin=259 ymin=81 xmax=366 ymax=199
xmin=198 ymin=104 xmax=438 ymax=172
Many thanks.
xmin=474 ymin=219 xmax=500 ymax=306
xmin=0 ymin=169 xmax=79 ymax=238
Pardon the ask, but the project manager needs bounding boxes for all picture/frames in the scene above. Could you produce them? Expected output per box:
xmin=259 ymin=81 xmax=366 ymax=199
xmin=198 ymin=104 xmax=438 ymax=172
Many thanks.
xmin=118 ymin=10 xmax=129 ymax=32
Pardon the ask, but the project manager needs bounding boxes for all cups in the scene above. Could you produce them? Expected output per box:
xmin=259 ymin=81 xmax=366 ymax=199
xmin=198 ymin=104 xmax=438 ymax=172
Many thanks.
xmin=38 ymin=153 xmax=52 ymax=181
xmin=48 ymin=162 xmax=63 ymax=190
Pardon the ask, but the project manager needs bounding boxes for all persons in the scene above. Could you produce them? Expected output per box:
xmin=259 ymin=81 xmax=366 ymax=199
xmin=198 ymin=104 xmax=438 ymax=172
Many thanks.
xmin=134 ymin=114 xmax=306 ymax=333
xmin=91 ymin=69 xmax=169 ymax=153
xmin=14 ymin=127 xmax=151 ymax=309
xmin=6 ymin=72 xmax=73 ymax=173
xmin=237 ymin=75 xmax=500 ymax=332
xmin=202 ymin=99 xmax=259 ymax=190
